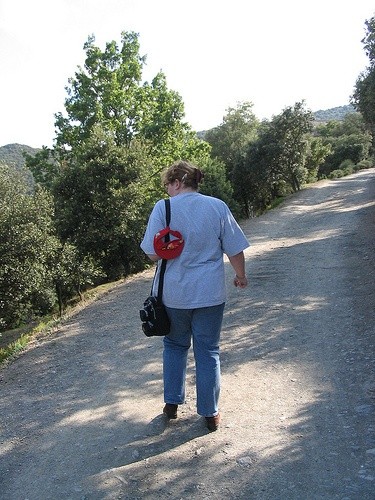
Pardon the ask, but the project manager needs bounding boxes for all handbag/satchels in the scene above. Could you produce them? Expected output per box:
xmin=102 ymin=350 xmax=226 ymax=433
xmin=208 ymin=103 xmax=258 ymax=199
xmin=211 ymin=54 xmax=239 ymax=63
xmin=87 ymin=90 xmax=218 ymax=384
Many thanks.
xmin=139 ymin=296 xmax=171 ymax=337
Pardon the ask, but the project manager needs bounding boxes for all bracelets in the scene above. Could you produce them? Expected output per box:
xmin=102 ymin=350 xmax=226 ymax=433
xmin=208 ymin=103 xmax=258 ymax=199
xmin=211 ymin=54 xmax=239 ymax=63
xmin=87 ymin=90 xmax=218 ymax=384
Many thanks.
xmin=235 ymin=273 xmax=246 ymax=280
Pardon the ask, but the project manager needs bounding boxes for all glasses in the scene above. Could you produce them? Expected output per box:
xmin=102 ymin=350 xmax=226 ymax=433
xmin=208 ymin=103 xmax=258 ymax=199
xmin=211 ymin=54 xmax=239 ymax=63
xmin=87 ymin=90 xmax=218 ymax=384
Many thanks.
xmin=164 ymin=179 xmax=175 ymax=187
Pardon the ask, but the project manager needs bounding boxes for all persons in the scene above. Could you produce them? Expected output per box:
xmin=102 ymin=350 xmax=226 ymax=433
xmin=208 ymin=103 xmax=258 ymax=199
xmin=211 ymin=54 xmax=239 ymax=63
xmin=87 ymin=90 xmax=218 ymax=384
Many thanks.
xmin=140 ymin=161 xmax=248 ymax=431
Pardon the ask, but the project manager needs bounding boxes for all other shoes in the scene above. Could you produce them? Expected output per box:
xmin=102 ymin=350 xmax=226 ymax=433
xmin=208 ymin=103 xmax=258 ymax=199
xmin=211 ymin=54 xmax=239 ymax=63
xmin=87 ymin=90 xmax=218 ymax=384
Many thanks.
xmin=205 ymin=412 xmax=220 ymax=432
xmin=163 ymin=404 xmax=178 ymax=419
